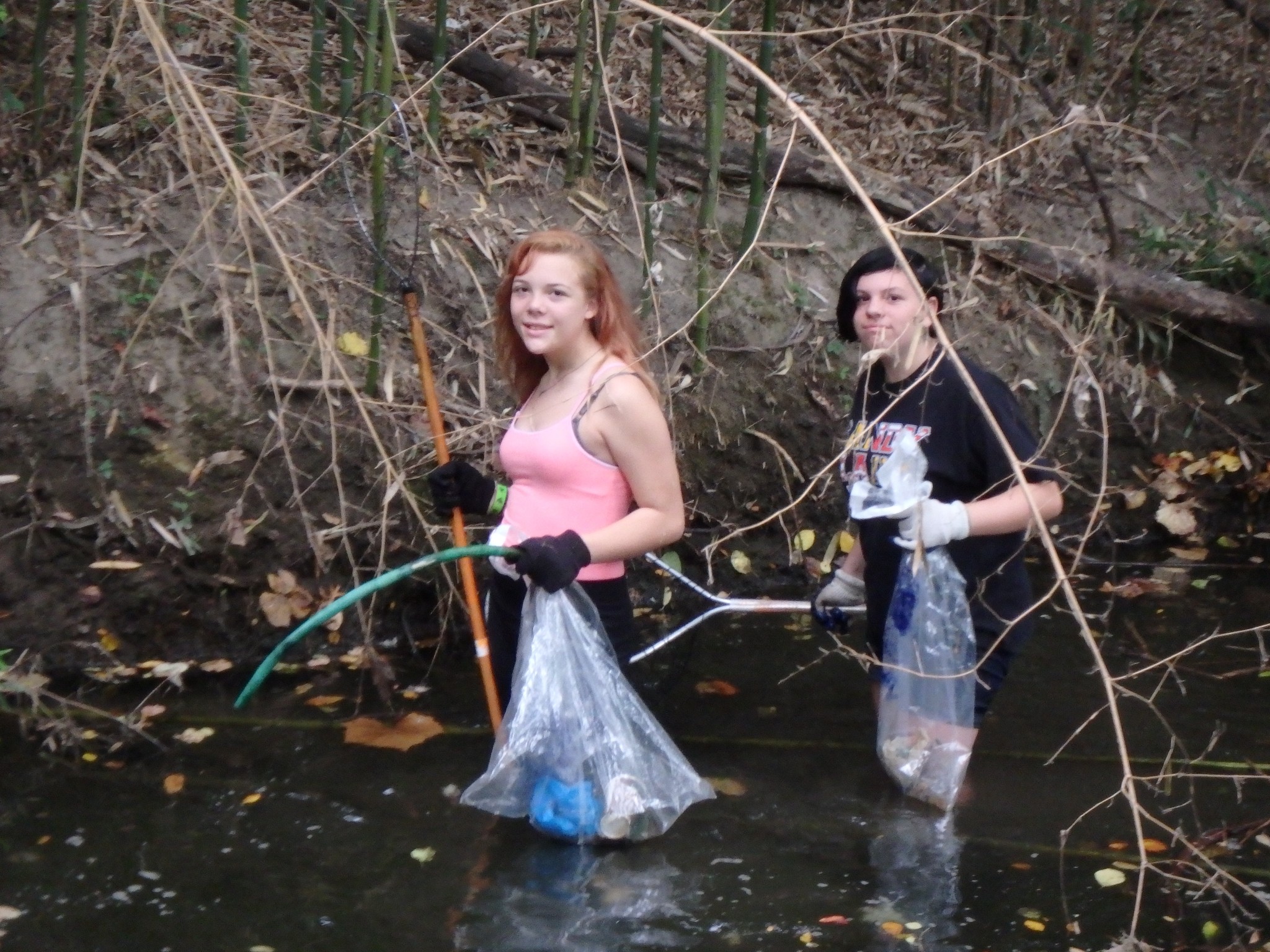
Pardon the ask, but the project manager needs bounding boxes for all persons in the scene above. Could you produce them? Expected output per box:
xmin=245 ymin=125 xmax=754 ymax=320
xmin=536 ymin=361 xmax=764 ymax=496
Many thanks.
xmin=814 ymin=246 xmax=1064 ymax=810
xmin=426 ymin=227 xmax=686 ymax=854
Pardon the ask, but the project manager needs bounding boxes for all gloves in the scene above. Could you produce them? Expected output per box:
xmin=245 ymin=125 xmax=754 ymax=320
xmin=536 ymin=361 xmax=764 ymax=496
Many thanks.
xmin=885 ymin=498 xmax=970 ymax=551
xmin=504 ymin=529 xmax=592 ymax=594
xmin=429 ymin=458 xmax=497 ymax=528
xmin=810 ymin=568 xmax=866 ymax=635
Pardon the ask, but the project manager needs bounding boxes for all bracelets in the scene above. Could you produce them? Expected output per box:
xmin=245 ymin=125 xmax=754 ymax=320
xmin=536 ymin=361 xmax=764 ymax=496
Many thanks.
xmin=488 ymin=483 xmax=508 ymax=517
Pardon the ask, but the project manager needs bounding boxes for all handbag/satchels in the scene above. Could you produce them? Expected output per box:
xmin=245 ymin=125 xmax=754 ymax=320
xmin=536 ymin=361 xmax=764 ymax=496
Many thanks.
xmin=875 ymin=548 xmax=978 ymax=813
xmin=459 ymin=522 xmax=718 ymax=842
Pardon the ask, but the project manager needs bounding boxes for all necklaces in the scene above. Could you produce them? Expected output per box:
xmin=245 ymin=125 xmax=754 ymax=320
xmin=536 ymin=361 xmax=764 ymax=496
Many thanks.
xmin=536 ymin=346 xmax=604 ymax=398
